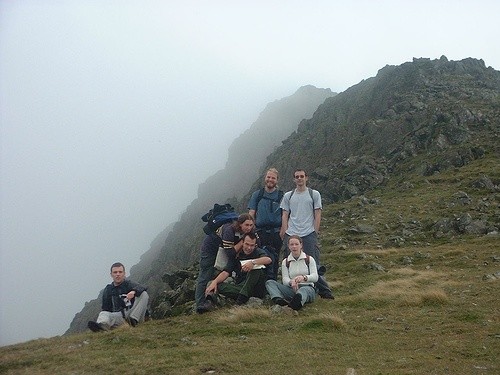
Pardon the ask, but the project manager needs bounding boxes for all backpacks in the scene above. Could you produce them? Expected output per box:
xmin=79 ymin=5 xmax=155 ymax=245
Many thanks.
xmin=201 ymin=204 xmax=239 ymax=243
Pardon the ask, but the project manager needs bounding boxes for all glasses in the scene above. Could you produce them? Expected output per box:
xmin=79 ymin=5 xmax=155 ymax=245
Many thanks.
xmin=295 ymin=175 xmax=305 ymax=179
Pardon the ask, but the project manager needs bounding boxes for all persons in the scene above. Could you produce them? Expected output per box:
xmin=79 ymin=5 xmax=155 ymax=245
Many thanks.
xmin=247 ymin=168 xmax=285 ymax=282
xmin=280 ymin=168 xmax=335 ymax=299
xmin=195 ymin=213 xmax=259 ymax=314
xmin=196 ymin=232 xmax=272 ymax=314
xmin=265 ymin=235 xmax=319 ymax=311
xmin=87 ymin=263 xmax=149 ymax=333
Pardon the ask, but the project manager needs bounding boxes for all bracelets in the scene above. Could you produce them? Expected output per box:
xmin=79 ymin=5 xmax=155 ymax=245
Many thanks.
xmin=302 ymin=275 xmax=307 ymax=282
xmin=251 ymin=259 xmax=256 ymax=265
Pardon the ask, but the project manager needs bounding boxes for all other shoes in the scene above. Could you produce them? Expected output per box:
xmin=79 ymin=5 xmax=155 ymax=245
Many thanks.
xmin=274 ymin=298 xmax=288 ymax=306
xmin=290 ymin=293 xmax=301 ymax=309
xmin=321 ymin=294 xmax=334 ymax=299
xmin=88 ymin=321 xmax=107 ymax=331
xmin=196 ymin=304 xmax=214 ymax=312
xmin=127 ymin=317 xmax=137 ymax=326
xmin=237 ymin=296 xmax=246 ymax=304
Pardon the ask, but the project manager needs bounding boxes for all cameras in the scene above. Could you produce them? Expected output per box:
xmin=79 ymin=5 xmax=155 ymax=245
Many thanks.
xmin=124 ymin=298 xmax=132 ymax=307
